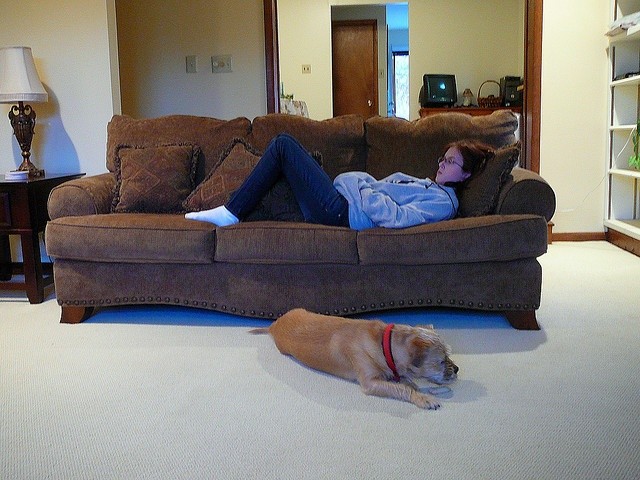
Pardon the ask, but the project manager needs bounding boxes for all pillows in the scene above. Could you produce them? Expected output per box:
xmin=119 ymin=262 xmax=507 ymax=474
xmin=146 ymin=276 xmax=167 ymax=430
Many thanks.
xmin=110 ymin=140 xmax=200 ymax=215
xmin=182 ymin=137 xmax=324 ymax=223
xmin=456 ymin=139 xmax=522 ymax=217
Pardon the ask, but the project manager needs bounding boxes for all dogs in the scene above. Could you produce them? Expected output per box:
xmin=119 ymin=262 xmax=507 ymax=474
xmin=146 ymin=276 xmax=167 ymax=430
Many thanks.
xmin=246 ymin=307 xmax=460 ymax=411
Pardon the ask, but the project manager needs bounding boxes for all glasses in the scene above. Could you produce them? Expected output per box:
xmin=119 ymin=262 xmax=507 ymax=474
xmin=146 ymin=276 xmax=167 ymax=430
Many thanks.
xmin=437 ymin=157 xmax=464 ymax=169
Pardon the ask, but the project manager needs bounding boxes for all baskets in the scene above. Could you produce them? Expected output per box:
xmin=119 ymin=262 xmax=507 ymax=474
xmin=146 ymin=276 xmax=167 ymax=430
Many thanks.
xmin=478 ymin=79 xmax=502 ymax=107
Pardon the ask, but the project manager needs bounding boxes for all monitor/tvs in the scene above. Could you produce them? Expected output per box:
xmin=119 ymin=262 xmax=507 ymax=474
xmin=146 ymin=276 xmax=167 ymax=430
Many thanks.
xmin=418 ymin=73 xmax=457 ymax=108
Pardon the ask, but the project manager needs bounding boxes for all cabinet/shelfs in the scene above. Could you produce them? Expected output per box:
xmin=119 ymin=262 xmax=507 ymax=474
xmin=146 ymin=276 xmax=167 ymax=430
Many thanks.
xmin=419 ymin=105 xmax=522 ymax=119
xmin=602 ymin=0 xmax=640 ymax=256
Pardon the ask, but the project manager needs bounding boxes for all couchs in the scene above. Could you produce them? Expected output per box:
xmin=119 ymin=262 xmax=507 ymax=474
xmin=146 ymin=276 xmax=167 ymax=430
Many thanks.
xmin=44 ymin=108 xmax=556 ymax=331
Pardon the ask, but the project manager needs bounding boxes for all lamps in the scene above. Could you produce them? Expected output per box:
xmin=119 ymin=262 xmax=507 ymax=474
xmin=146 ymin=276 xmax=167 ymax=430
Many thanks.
xmin=0 ymin=45 xmax=49 ymax=179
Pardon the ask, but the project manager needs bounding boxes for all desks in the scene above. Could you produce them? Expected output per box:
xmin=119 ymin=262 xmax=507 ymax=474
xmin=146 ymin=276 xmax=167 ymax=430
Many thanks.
xmin=280 ymin=100 xmax=310 ymax=121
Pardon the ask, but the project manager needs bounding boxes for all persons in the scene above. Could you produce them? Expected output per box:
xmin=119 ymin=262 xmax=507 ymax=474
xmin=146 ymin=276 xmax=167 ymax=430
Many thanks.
xmin=184 ymin=133 xmax=495 ymax=232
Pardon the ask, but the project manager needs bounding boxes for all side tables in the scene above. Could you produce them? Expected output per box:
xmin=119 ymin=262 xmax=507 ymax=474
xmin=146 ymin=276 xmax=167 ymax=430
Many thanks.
xmin=0 ymin=172 xmax=87 ymax=304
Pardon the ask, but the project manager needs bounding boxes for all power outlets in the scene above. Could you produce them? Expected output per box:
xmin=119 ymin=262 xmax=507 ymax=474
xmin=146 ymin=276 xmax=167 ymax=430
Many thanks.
xmin=301 ymin=64 xmax=311 ymax=74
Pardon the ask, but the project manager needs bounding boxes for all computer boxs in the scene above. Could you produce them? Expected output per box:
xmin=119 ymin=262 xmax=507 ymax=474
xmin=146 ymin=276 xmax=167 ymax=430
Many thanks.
xmin=500 ymin=76 xmax=521 ymax=107
